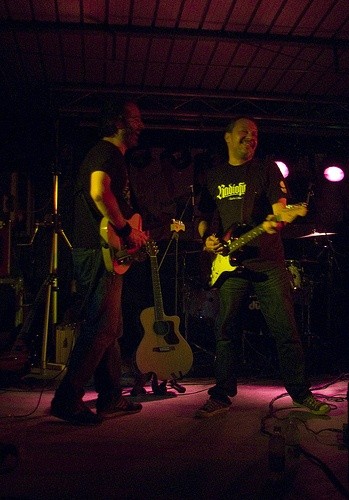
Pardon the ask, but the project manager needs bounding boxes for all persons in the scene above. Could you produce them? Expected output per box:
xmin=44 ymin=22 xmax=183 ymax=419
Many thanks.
xmin=48 ymin=99 xmax=148 ymax=427
xmin=192 ymin=117 xmax=331 ymax=417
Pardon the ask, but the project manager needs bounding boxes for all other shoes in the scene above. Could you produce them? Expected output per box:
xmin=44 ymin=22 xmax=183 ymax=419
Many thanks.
xmin=96 ymin=396 xmax=143 ymax=418
xmin=291 ymin=392 xmax=330 ymax=415
xmin=49 ymin=398 xmax=102 ymax=426
xmin=195 ymin=397 xmax=230 ymax=418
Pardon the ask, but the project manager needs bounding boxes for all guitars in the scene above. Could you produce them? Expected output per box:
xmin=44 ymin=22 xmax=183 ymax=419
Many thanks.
xmin=199 ymin=201 xmax=308 ymax=287
xmin=99 ymin=213 xmax=185 ymax=276
xmin=134 ymin=240 xmax=193 ymax=383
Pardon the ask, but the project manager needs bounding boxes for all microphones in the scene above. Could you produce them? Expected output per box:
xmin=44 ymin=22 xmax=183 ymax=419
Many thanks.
xmin=191 ymin=185 xmax=195 ymax=206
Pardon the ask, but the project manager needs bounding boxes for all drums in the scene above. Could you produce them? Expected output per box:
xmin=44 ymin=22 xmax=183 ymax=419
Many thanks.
xmin=249 ymin=259 xmax=302 ymax=301
xmin=178 ymin=283 xmax=219 ymax=325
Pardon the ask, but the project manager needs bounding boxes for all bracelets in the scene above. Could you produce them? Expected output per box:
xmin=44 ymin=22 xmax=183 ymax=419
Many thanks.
xmin=200 ymin=229 xmax=213 ymax=245
xmin=115 ymin=221 xmax=132 ymax=239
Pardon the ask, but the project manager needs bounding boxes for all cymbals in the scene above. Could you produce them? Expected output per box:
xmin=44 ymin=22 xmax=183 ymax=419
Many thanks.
xmin=164 ymin=249 xmax=202 ymax=255
xmin=293 ymin=229 xmax=339 ymax=239
